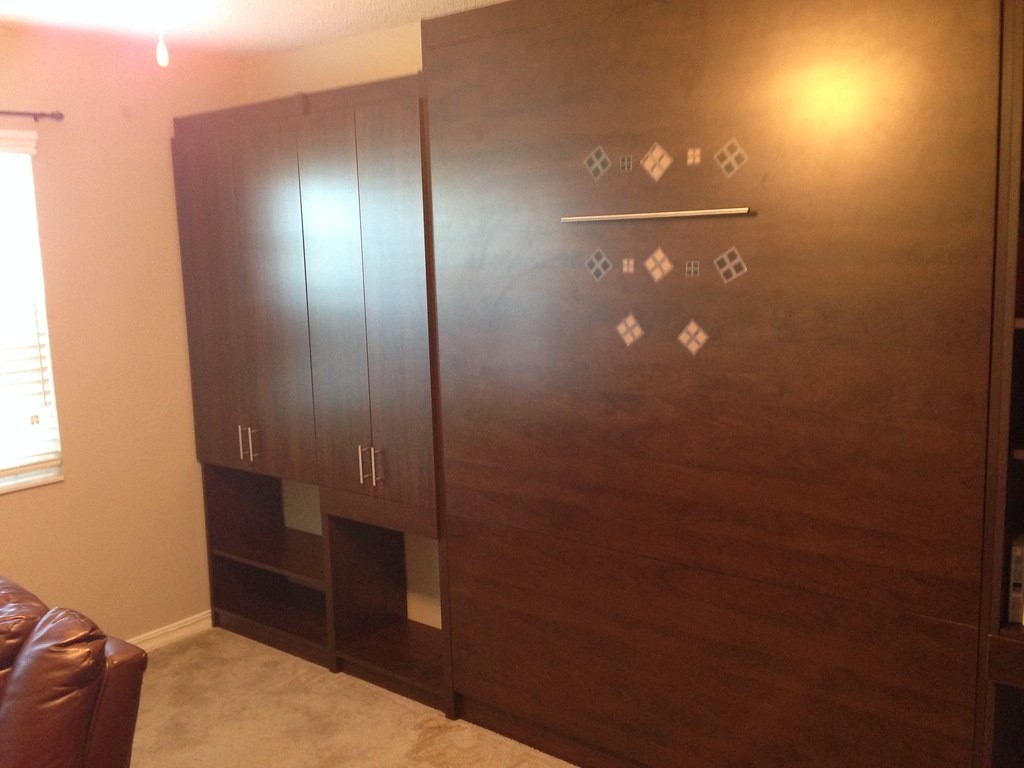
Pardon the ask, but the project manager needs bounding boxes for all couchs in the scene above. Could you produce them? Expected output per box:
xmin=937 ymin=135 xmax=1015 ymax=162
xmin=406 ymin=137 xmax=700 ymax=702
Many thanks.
xmin=0 ymin=573 xmax=148 ymax=768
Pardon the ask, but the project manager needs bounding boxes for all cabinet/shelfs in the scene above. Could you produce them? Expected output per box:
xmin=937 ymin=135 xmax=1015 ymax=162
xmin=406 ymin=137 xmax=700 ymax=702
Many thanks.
xmin=420 ymin=0 xmax=1024 ymax=768
xmin=170 ymin=95 xmax=321 ymax=487
xmin=294 ymin=74 xmax=440 ymax=507
xmin=201 ymin=464 xmax=448 ymax=713
